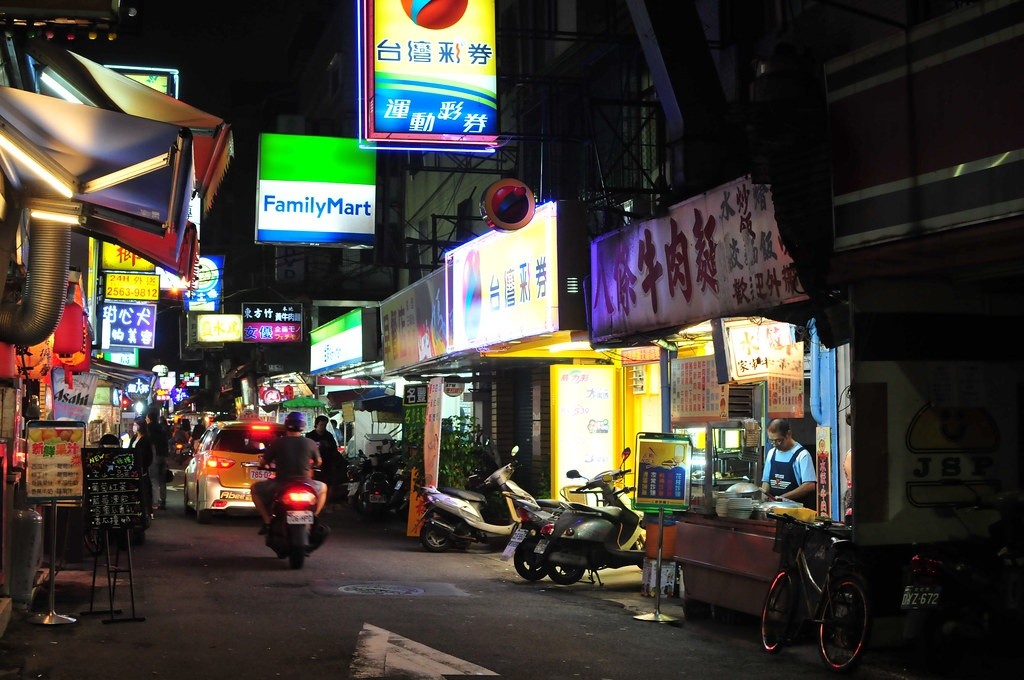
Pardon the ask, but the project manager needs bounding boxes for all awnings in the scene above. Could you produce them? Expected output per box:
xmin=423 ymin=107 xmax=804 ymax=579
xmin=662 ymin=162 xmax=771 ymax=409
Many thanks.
xmin=27 ymin=41 xmax=235 ymax=287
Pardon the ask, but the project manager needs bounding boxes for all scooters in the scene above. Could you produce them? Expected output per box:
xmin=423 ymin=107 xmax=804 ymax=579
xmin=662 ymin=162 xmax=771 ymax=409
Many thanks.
xmin=339 ymin=433 xmax=657 ymax=586
xmin=163 ymin=420 xmax=206 ymax=467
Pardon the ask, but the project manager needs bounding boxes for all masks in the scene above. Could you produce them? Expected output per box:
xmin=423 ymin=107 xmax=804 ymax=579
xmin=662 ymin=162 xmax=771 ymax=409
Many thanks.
xmin=132 ymin=426 xmax=138 ymax=432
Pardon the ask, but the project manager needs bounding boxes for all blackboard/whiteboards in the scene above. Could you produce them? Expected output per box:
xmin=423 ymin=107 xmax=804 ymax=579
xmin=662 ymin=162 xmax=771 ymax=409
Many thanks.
xmin=80 ymin=446 xmax=149 ymax=530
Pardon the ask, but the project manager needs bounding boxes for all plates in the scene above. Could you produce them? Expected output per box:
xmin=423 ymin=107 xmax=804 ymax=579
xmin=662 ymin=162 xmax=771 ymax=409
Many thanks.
xmin=715 ymin=489 xmax=755 ymax=519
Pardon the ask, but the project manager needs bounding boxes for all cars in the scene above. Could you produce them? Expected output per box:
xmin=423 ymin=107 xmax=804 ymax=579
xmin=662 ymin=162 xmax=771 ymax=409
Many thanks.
xmin=184 ymin=419 xmax=295 ymax=525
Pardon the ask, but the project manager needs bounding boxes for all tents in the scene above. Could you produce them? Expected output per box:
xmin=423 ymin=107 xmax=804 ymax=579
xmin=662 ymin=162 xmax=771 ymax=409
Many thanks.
xmin=280 ymin=396 xmax=326 ymax=415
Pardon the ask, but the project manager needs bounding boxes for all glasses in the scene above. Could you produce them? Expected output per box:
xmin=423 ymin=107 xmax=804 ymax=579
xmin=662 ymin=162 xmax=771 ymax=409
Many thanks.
xmin=145 ymin=418 xmax=149 ymax=420
xmin=767 ymin=437 xmax=786 ymax=446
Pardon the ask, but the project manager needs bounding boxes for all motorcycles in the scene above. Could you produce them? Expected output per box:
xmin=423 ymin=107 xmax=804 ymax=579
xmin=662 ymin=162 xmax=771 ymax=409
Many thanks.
xmin=900 ymin=487 xmax=1024 ymax=677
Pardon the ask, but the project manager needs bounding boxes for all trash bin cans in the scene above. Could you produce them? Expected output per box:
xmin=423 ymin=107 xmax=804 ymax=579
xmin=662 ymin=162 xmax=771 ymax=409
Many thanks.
xmin=8 ymin=508 xmax=45 ymax=610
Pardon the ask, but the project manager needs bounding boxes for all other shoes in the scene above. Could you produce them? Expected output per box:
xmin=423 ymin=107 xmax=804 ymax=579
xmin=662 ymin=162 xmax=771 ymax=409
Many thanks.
xmin=257 ymin=523 xmax=275 ymax=534
xmin=152 ymin=498 xmax=166 ymax=509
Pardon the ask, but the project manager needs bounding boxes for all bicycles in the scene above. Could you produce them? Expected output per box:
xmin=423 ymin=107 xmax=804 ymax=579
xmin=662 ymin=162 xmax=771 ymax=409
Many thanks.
xmin=761 ymin=511 xmax=868 ymax=673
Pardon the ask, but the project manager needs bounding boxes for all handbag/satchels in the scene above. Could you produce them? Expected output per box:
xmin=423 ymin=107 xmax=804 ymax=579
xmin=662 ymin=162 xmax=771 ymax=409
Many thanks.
xmin=165 ymin=470 xmax=174 ymax=483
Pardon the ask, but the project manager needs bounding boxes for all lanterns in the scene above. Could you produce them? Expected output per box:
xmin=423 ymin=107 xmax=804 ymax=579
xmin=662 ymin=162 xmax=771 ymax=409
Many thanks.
xmin=13 ymin=283 xmax=88 ymax=391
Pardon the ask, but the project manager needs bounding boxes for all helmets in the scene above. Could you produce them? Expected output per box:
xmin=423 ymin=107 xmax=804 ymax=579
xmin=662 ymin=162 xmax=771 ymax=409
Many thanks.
xmin=284 ymin=411 xmax=306 ymax=432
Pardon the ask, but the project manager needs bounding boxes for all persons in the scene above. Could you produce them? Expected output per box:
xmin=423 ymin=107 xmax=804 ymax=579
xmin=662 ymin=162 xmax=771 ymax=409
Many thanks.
xmin=330 ymin=419 xmax=343 ymax=443
xmin=761 ymin=418 xmax=817 ymax=501
xmin=144 ymin=412 xmax=169 ymax=508
xmin=128 ymin=417 xmax=154 ymax=521
xmin=306 ymin=415 xmax=339 ymax=483
xmin=160 ymin=414 xmax=207 ymax=460
xmin=339 ymin=414 xmax=353 ymax=441
xmin=252 ymin=411 xmax=326 ymax=535
